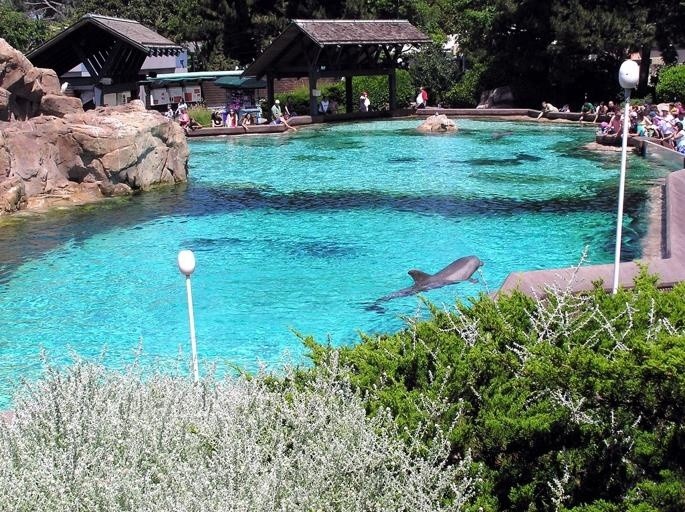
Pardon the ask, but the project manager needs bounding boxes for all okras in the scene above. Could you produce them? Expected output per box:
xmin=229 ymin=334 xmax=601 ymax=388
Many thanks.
xmin=375 ymin=255 xmax=484 ymax=302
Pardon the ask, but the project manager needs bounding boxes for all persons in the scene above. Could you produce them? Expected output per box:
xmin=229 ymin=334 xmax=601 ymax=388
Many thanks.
xmin=163 ymin=97 xmax=344 ymax=131
xmin=579 ymin=101 xmax=685 ymax=153
xmin=536 ymin=102 xmax=569 ymax=120
xmin=412 ymin=87 xmax=427 ymax=119
xmin=360 ymin=91 xmax=372 ymax=114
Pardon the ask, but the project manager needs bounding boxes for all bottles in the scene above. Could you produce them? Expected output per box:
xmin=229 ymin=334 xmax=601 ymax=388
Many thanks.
xmin=275 ymin=99 xmax=280 ymax=104
xmin=649 ymin=112 xmax=656 ymax=116
xmin=632 ymin=112 xmax=638 ymax=117
xmin=661 ymin=105 xmax=669 ymax=111
